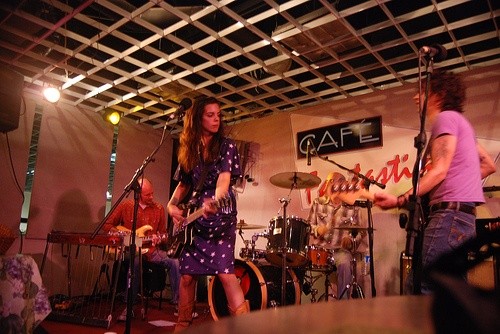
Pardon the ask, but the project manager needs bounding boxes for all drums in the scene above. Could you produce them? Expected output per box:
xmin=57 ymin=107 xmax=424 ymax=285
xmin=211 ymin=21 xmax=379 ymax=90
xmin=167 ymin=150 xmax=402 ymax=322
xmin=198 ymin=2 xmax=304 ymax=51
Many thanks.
xmin=209 ymin=258 xmax=300 ymax=322
xmin=264 ymin=215 xmax=312 ymax=267
xmin=307 ymin=244 xmax=337 ymax=272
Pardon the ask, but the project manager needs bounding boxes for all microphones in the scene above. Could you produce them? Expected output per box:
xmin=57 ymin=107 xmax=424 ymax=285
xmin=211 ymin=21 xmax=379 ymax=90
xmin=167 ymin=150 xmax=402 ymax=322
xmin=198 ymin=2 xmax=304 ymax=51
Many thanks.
xmin=307 ymin=140 xmax=311 ymax=165
xmin=169 ymin=98 xmax=193 ymax=120
xmin=420 ymin=43 xmax=447 ymax=63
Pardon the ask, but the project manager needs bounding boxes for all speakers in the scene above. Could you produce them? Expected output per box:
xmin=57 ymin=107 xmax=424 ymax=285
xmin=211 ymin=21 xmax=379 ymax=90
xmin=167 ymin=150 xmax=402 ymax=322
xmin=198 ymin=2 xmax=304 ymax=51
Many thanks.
xmin=0 ymin=67 xmax=24 ymax=134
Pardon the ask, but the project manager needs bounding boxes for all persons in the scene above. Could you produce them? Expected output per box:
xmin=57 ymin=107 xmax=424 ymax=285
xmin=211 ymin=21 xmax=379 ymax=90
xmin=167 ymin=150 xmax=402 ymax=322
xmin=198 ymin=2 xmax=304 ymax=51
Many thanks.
xmin=370 ymin=69 xmax=498 ymax=296
xmin=164 ymin=95 xmax=250 ymax=334
xmin=102 ymin=176 xmax=200 ymax=323
xmin=305 ymin=171 xmax=367 ymax=300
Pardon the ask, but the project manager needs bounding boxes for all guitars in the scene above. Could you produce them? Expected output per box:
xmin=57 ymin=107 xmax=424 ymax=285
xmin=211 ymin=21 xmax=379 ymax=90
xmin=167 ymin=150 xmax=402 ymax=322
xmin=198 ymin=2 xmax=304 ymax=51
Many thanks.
xmin=104 ymin=225 xmax=169 ymax=260
xmin=328 ymin=180 xmax=426 ymax=232
xmin=167 ymin=194 xmax=232 ymax=259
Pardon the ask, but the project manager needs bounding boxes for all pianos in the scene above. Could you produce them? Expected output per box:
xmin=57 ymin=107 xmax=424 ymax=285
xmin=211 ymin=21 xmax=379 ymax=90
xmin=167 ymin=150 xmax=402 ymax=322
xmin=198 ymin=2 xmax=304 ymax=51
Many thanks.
xmin=47 ymin=230 xmax=143 ymax=248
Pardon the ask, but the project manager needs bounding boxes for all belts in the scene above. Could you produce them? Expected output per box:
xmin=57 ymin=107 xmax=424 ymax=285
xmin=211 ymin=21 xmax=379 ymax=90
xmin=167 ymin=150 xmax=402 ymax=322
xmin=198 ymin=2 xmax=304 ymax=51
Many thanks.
xmin=429 ymin=202 xmax=476 ymax=216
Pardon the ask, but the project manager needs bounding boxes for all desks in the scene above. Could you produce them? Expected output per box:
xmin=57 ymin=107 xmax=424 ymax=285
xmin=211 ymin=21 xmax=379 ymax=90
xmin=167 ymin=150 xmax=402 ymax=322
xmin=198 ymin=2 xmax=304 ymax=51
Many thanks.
xmin=0 ymin=252 xmax=34 ymax=334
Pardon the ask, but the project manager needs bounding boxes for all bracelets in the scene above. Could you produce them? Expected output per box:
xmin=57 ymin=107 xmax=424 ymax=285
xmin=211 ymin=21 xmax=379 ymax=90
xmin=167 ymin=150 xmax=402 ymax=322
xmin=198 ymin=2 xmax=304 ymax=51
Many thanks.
xmin=396 ymin=194 xmax=409 ymax=210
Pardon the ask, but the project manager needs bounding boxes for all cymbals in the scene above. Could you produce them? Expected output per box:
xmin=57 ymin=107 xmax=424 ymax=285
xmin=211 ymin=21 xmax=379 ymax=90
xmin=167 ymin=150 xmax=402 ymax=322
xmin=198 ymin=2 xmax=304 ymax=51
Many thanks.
xmin=269 ymin=172 xmax=321 ymax=189
xmin=236 ymin=223 xmax=266 ymax=229
xmin=331 ymin=226 xmax=377 ymax=231
